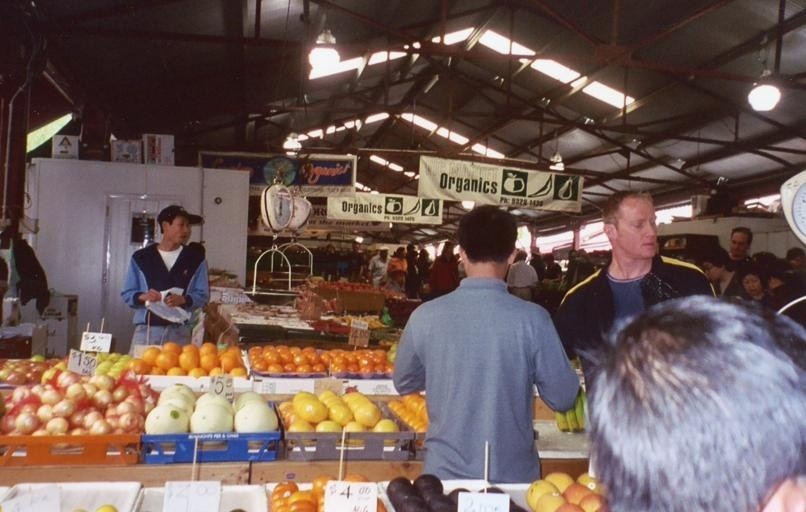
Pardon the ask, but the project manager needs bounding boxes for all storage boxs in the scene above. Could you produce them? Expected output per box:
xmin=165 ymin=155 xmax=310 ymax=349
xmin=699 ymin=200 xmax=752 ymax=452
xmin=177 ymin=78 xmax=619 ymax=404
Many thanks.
xmin=134 ymin=344 xmax=252 ymax=392
xmin=133 ymin=487 xmax=265 ymax=512
xmin=501 ymin=484 xmax=536 ymax=512
xmin=379 ymin=479 xmax=524 ymax=512
xmin=1 ymin=483 xmax=142 ymax=512
xmin=252 ymin=370 xmax=327 ymax=394
xmin=331 ymin=372 xmax=398 ymax=395
xmin=319 ymin=284 xmax=384 ymax=315
xmin=265 ymin=481 xmax=389 ymax=512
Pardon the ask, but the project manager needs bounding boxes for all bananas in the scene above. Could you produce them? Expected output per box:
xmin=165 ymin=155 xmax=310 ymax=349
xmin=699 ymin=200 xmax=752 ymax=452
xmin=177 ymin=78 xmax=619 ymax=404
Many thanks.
xmin=553 ymin=388 xmax=586 ymax=432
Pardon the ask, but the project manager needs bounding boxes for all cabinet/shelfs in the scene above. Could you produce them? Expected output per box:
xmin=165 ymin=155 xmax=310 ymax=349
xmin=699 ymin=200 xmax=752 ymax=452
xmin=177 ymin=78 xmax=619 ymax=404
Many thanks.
xmin=43 ymin=295 xmax=78 ymax=357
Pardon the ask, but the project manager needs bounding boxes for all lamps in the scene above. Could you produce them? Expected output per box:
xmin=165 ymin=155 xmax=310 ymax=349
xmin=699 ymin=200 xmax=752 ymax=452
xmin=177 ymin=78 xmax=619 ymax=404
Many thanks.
xmin=748 ymin=43 xmax=780 ymax=111
xmin=549 ymin=138 xmax=564 ymax=171
xmin=284 ymin=135 xmax=302 ymax=155
xmin=309 ymin=28 xmax=339 ymax=70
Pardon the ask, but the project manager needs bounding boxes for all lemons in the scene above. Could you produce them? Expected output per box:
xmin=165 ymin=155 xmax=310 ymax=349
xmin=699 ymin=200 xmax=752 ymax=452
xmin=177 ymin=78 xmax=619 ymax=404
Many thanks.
xmin=282 ymin=391 xmax=401 ymax=451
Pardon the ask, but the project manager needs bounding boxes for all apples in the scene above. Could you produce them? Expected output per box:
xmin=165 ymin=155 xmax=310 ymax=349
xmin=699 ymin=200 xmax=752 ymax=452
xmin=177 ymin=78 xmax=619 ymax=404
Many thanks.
xmin=528 ymin=472 xmax=609 ymax=512
xmin=0 ymin=352 xmax=133 ymax=384
xmin=94 ymin=505 xmax=118 ymax=511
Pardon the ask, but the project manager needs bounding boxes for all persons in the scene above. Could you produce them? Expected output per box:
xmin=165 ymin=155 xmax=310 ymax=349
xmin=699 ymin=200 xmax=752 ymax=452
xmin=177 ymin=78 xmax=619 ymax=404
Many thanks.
xmin=585 ymin=293 xmax=805 ymax=512
xmin=121 ymin=206 xmax=211 ymax=354
xmin=556 ymin=191 xmax=718 ymax=364
xmin=395 ymin=206 xmax=581 ymax=481
xmin=507 ymin=247 xmax=593 ymax=301
xmin=325 ymin=239 xmax=429 ymax=299
xmin=428 ymin=240 xmax=460 ymax=293
xmin=705 ymin=226 xmax=806 ymax=315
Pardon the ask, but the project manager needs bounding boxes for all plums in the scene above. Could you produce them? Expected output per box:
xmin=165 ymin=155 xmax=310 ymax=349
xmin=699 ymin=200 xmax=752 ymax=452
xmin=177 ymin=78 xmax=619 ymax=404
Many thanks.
xmin=388 ymin=473 xmax=528 ymax=511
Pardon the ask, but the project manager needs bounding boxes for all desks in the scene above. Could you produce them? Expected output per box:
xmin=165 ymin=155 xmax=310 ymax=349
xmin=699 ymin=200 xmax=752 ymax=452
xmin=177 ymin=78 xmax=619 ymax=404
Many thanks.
xmin=251 ymin=394 xmax=422 ymax=484
xmin=0 ymin=392 xmax=250 ymax=485
xmin=0 ymin=336 xmax=32 ymax=359
xmin=535 ymin=395 xmax=591 ymax=478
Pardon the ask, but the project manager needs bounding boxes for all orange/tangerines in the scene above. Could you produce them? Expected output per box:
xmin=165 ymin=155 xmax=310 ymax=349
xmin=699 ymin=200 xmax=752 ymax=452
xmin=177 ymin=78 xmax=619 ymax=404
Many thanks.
xmin=250 ymin=344 xmax=324 ymax=373
xmin=321 ymin=349 xmax=393 ymax=373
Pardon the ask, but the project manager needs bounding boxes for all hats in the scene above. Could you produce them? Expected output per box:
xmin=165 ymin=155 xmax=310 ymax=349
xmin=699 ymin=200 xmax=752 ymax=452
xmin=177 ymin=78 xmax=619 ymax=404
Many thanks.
xmin=157 ymin=206 xmax=203 ymax=224
